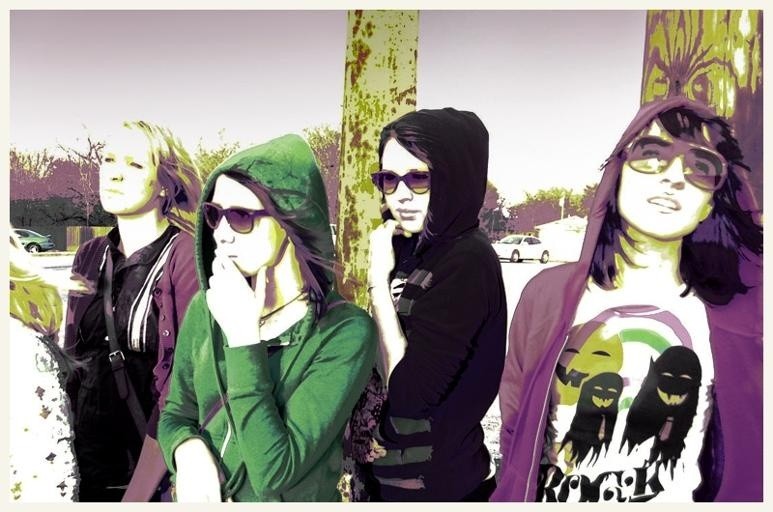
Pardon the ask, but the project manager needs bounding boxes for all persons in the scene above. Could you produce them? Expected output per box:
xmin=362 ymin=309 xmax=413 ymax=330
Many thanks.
xmin=489 ymin=97 xmax=761 ymax=501
xmin=345 ymin=108 xmax=507 ymax=502
xmin=8 ymin=217 xmax=94 ymax=502
xmin=63 ymin=120 xmax=197 ymax=503
xmin=156 ymin=133 xmax=378 ymax=503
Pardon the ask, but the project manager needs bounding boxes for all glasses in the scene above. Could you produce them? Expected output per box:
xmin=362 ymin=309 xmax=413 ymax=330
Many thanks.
xmin=627 ymin=136 xmax=727 ymax=190
xmin=371 ymin=170 xmax=432 ymax=194
xmin=202 ymin=202 xmax=270 ymax=234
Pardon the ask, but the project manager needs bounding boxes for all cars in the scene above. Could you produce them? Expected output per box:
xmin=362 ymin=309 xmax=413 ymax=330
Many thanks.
xmin=12 ymin=228 xmax=55 ymax=254
xmin=490 ymin=234 xmax=551 ymax=264
